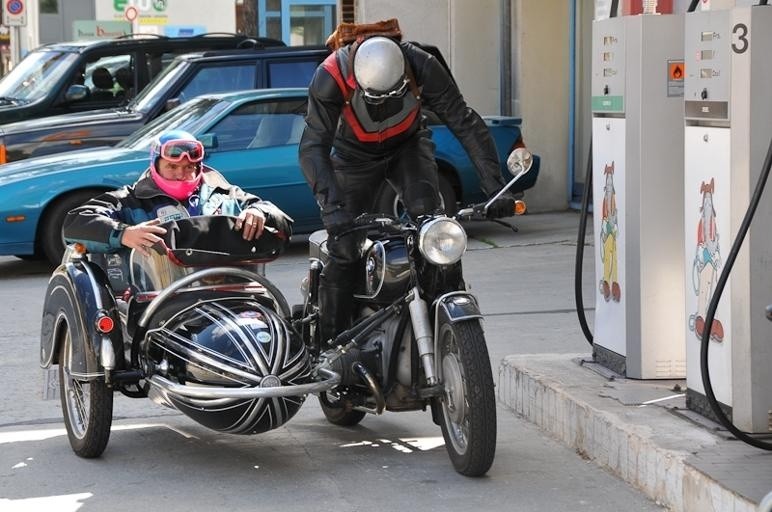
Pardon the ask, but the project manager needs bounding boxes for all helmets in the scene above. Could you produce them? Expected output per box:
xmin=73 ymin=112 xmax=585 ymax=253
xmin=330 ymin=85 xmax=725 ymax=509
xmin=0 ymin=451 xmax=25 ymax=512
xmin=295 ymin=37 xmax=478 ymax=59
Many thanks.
xmin=150 ymin=130 xmax=202 ymax=201
xmin=354 ymin=36 xmax=405 ymax=95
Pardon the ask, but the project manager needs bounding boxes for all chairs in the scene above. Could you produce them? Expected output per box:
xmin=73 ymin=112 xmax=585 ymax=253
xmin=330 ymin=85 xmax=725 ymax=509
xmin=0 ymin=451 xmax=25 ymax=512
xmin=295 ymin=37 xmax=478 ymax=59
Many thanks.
xmin=91 ymin=67 xmax=114 ymax=101
xmin=286 ymin=113 xmax=307 ymax=145
xmin=245 ymin=117 xmax=279 ymax=149
xmin=113 ymin=67 xmax=135 ymax=103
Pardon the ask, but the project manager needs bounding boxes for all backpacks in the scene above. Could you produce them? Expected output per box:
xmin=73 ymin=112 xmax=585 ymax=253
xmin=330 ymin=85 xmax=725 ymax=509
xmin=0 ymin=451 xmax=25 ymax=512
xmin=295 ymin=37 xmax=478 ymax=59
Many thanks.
xmin=325 ymin=17 xmax=421 ymax=105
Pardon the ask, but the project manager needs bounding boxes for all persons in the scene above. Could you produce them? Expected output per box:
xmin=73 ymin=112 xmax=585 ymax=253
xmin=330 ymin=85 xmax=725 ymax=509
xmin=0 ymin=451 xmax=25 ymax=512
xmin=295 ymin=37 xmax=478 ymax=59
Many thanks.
xmin=61 ymin=129 xmax=294 ymax=257
xmin=299 ymin=37 xmax=515 ymax=351
xmin=599 ymin=161 xmax=621 ymax=303
xmin=689 ymin=180 xmax=726 ymax=343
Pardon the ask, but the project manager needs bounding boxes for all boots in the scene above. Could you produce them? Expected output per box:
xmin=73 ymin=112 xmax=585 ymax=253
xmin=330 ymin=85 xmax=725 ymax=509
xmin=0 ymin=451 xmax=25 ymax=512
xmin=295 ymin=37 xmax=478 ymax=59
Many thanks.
xmin=318 ymin=285 xmax=353 ymax=350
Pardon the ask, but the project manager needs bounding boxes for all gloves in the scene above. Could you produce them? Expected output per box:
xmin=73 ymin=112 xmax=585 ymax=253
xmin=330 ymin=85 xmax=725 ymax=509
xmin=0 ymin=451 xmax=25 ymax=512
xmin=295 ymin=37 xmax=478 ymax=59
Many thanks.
xmin=326 ymin=222 xmax=358 ymax=262
xmin=480 ymin=176 xmax=514 ymax=218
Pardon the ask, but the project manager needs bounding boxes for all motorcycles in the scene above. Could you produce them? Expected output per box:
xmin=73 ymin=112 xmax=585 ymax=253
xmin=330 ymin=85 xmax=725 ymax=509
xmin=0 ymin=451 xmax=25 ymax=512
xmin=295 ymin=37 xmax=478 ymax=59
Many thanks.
xmin=37 ymin=148 xmax=542 ymax=483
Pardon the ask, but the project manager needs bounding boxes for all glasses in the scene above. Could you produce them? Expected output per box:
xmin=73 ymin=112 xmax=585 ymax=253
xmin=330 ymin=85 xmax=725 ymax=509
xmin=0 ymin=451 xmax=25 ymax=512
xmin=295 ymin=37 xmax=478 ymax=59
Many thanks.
xmin=364 ymin=79 xmax=410 ymax=105
xmin=161 ymin=139 xmax=205 ymax=162
